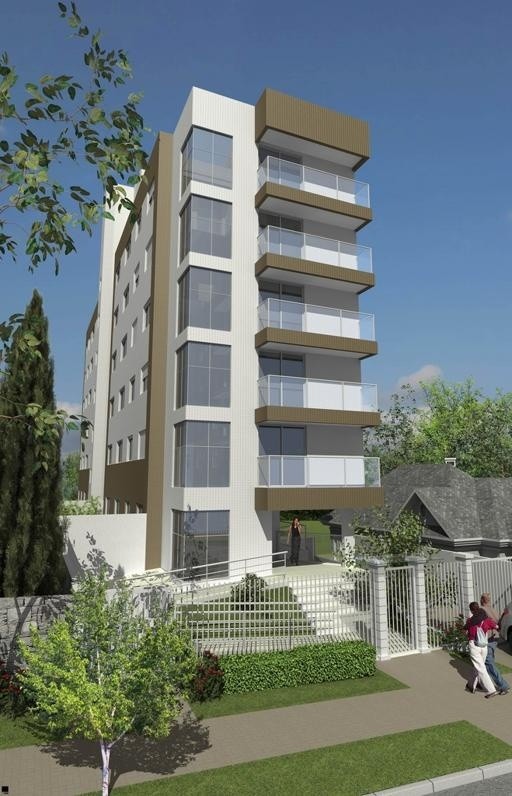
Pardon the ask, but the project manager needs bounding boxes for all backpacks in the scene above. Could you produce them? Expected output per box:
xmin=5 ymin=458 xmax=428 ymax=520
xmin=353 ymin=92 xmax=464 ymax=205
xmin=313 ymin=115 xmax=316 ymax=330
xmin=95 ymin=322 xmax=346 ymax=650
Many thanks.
xmin=474 ymin=626 xmax=488 ymax=648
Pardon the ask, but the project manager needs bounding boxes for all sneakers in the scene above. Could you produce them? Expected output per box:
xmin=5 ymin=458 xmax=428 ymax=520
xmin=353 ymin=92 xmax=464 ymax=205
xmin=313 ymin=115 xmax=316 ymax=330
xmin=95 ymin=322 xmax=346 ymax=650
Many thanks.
xmin=466 ymin=682 xmax=511 ymax=700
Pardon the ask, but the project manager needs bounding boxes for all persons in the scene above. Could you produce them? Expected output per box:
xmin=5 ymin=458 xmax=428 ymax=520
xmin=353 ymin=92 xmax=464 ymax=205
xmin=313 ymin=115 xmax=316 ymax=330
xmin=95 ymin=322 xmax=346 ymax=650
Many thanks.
xmin=469 ymin=592 xmax=511 ymax=698
xmin=464 ymin=600 xmax=499 ymax=700
xmin=286 ymin=516 xmax=303 ymax=567
